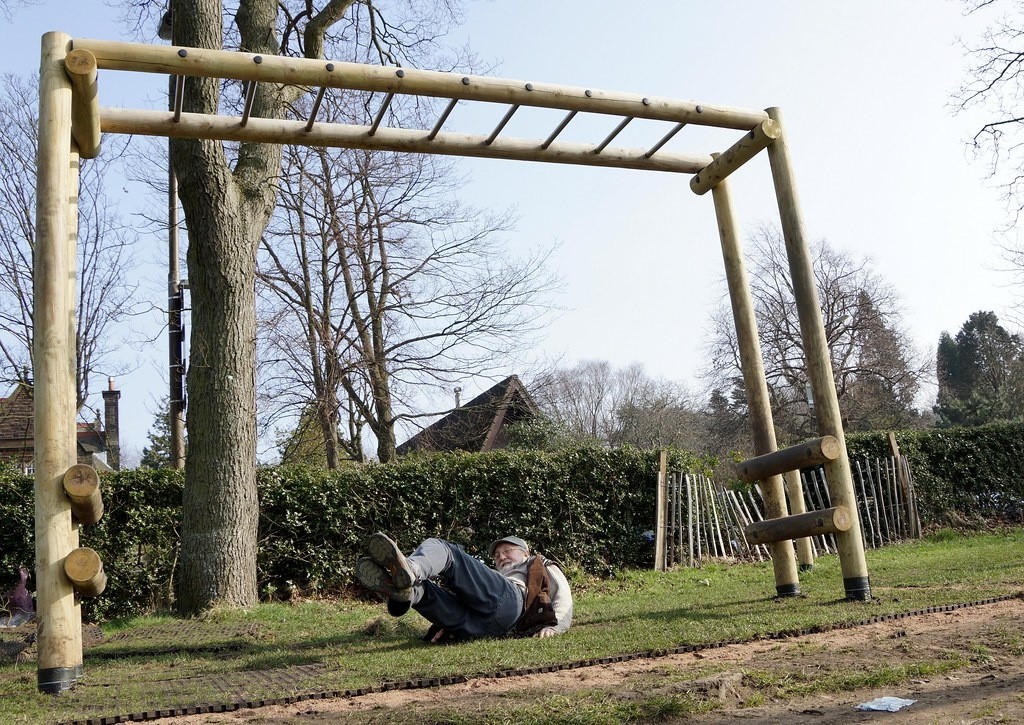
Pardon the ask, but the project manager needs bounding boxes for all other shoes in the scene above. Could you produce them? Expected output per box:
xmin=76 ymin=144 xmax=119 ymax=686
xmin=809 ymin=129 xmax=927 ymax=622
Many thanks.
xmin=355 ymin=557 xmax=414 ymax=602
xmin=367 ymin=532 xmax=417 ymax=590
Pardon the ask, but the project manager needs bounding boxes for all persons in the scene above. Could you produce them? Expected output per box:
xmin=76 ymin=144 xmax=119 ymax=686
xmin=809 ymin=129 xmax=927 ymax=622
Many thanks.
xmin=359 ymin=533 xmax=571 ymax=644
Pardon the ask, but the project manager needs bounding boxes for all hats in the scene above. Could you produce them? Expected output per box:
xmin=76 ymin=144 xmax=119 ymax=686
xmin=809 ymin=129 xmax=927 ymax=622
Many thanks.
xmin=487 ymin=536 xmax=529 ymax=558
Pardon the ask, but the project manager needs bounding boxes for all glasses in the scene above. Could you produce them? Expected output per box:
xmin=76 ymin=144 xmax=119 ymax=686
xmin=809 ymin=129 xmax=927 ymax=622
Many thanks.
xmin=493 ymin=547 xmax=522 ymax=560
